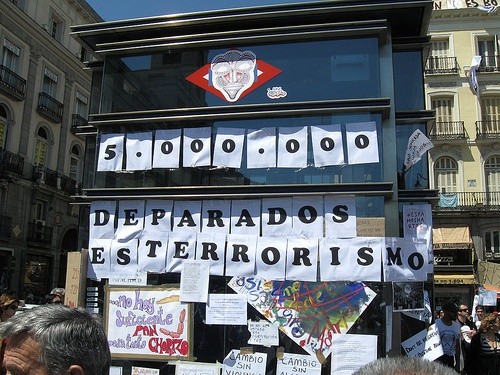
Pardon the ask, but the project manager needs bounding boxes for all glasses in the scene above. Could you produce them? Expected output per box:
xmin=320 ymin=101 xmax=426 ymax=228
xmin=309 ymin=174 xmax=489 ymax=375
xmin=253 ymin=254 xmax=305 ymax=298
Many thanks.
xmin=8 ymin=305 xmax=18 ymax=310
xmin=459 ymin=309 xmax=468 ymax=312
xmin=48 ymin=301 xmax=62 ymax=305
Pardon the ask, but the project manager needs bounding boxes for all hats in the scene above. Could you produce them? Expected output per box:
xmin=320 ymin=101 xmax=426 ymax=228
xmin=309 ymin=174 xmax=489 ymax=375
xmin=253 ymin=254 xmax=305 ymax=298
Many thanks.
xmin=442 ymin=302 xmax=463 ymax=312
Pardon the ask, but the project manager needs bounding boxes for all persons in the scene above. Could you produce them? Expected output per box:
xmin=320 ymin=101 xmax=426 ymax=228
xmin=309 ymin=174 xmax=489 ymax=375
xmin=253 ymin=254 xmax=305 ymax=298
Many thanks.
xmin=0 ymin=304 xmax=110 ymax=375
xmin=0 ymin=287 xmax=66 ymax=322
xmin=394 ymin=288 xmax=424 ymax=310
xmin=351 ymin=356 xmax=460 ymax=375
xmin=456 ymin=304 xmax=478 ymax=375
xmin=468 ymin=312 xmax=500 ymax=375
xmin=434 ymin=299 xmax=462 ymax=373
xmin=475 ymin=305 xmax=485 ymax=320
xmin=435 ymin=310 xmax=444 ymax=318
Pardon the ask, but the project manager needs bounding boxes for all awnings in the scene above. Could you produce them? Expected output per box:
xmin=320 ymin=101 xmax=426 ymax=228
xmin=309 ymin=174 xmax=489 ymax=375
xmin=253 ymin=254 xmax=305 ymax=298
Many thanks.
xmin=434 ymin=275 xmax=475 ymax=284
xmin=432 ymin=227 xmax=473 ymax=250
